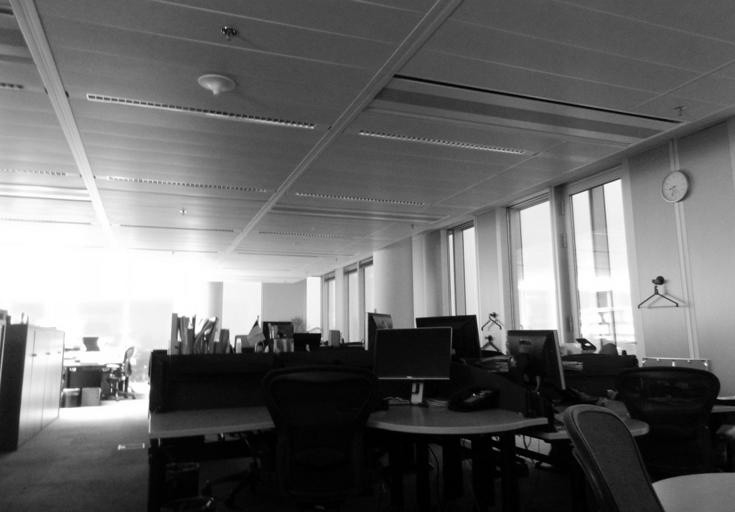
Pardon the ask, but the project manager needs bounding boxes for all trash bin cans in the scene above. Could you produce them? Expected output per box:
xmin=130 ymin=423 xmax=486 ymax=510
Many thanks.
xmin=64 ymin=388 xmax=81 ymax=408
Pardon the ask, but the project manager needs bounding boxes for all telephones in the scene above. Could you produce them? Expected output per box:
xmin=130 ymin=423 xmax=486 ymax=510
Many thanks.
xmin=449 ymin=385 xmax=501 ymax=412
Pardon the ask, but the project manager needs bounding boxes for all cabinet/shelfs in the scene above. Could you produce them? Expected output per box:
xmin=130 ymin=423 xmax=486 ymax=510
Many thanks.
xmin=3 ymin=324 xmax=64 ymax=451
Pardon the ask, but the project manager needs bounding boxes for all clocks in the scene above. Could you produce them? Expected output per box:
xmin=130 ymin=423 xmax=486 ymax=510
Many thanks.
xmin=660 ymin=170 xmax=690 ymax=202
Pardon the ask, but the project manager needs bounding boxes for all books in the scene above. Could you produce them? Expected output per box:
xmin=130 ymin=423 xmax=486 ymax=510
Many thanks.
xmin=169 ymin=311 xmax=230 ymax=354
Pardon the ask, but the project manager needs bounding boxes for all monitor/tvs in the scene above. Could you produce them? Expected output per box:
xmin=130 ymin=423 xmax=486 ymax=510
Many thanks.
xmin=375 ymin=326 xmax=452 ymax=405
xmin=367 ymin=311 xmax=392 ymax=351
xmin=507 ymin=329 xmax=566 ymax=390
xmin=416 ymin=314 xmax=480 ymax=360
xmin=262 ymin=321 xmax=294 ymax=340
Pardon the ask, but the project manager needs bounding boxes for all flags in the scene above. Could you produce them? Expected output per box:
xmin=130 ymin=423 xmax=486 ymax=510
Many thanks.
xmin=246 ymin=319 xmax=264 ymax=347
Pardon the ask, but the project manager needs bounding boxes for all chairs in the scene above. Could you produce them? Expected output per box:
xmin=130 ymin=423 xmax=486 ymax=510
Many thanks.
xmin=257 ymin=365 xmax=394 ymax=512
xmin=61 ymin=335 xmax=137 ymax=405
xmin=613 ymin=367 xmax=721 ymax=472
xmin=564 ymin=404 xmax=665 ymax=511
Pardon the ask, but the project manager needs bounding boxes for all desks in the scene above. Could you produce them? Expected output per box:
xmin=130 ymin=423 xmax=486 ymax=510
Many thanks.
xmin=652 ymin=469 xmax=735 ymax=510
xmin=538 ymin=407 xmax=649 ymax=439
xmin=147 ymin=399 xmax=548 ymax=512
